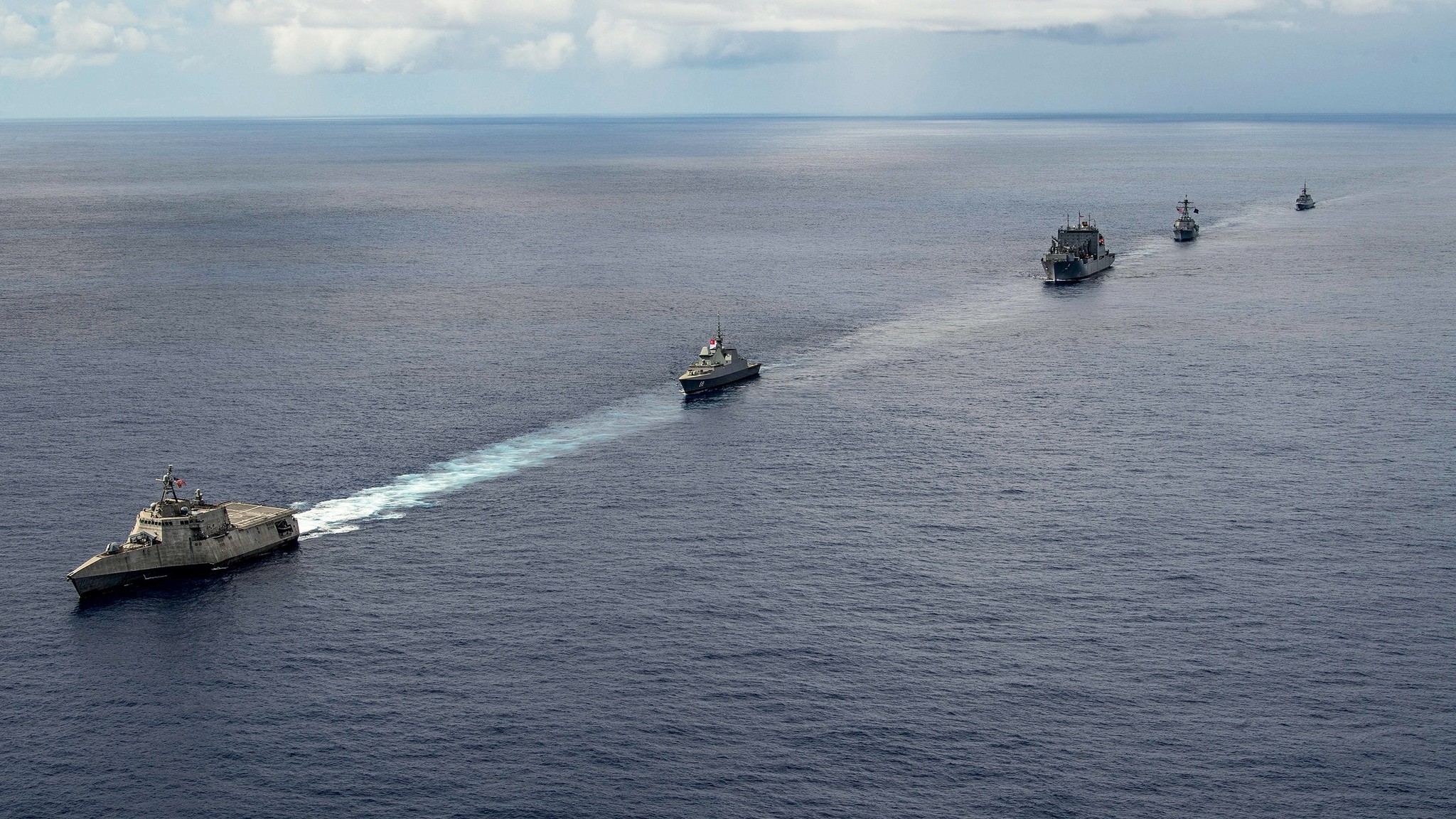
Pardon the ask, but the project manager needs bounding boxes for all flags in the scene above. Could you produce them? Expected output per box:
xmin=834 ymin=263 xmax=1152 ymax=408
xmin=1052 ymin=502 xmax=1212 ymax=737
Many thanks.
xmin=1177 ymin=208 xmax=1183 ymax=214
xmin=709 ymin=339 xmax=717 ymax=350
xmin=172 ymin=477 xmax=187 ymax=489
xmin=1193 ymin=208 xmax=1199 ymax=214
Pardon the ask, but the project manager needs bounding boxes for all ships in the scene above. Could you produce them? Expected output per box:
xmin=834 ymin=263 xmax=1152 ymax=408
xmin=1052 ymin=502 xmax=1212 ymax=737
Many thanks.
xmin=1040 ymin=210 xmax=1115 ymax=281
xmin=66 ymin=465 xmax=300 ymax=598
xmin=1295 ymin=179 xmax=1316 ymax=210
xmin=679 ymin=310 xmax=762 ymax=394
xmin=1174 ymin=194 xmax=1199 ymax=240
